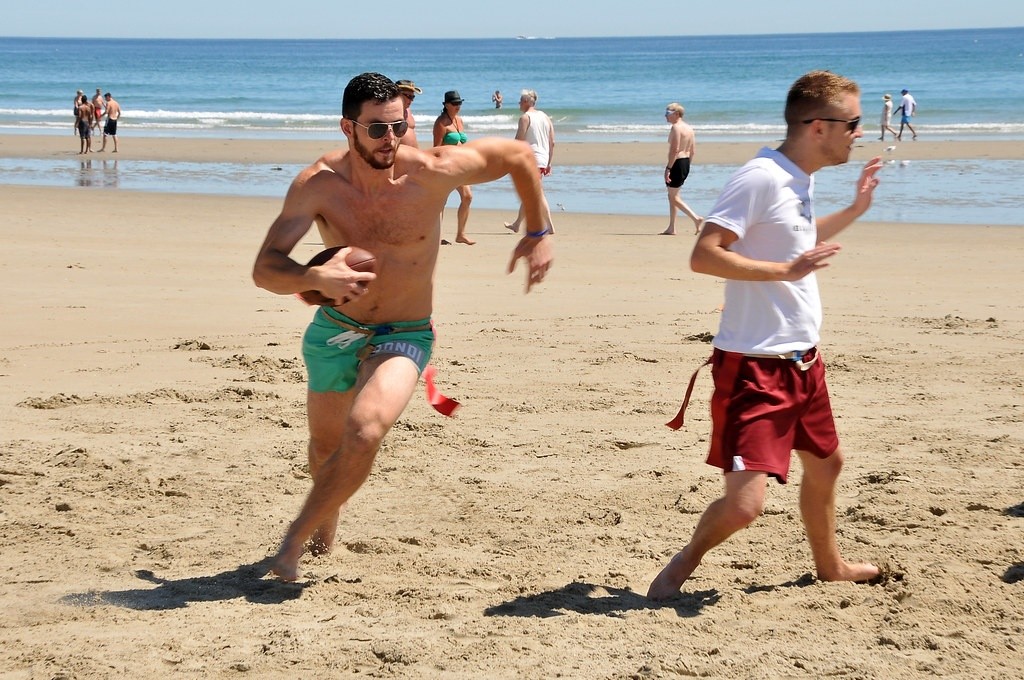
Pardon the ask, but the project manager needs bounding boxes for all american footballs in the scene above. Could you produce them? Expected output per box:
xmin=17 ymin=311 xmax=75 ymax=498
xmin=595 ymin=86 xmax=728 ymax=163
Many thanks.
xmin=298 ymin=245 xmax=376 ymax=306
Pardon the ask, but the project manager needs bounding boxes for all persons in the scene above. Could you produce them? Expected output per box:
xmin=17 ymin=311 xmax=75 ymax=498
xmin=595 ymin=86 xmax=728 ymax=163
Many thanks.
xmin=250 ymin=72 xmax=553 ymax=579
xmin=432 ymin=90 xmax=475 ymax=245
xmin=878 ymin=93 xmax=899 ymax=139
xmin=393 ymin=80 xmax=422 ymax=149
xmin=72 ymin=87 xmax=120 ymax=154
xmin=503 ymin=89 xmax=555 ymax=234
xmin=647 ymin=69 xmax=883 ymax=597
xmin=893 ymin=88 xmax=917 ymax=139
xmin=659 ymin=102 xmax=704 ymax=236
xmin=492 ymin=90 xmax=502 ymax=109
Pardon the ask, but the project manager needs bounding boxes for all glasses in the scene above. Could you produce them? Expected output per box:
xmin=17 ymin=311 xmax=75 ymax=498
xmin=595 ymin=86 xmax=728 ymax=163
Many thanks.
xmin=401 ymin=93 xmax=415 ymax=101
xmin=451 ymin=102 xmax=462 ymax=106
xmin=351 ymin=119 xmax=408 ymax=141
xmin=803 ymin=116 xmax=860 ymax=133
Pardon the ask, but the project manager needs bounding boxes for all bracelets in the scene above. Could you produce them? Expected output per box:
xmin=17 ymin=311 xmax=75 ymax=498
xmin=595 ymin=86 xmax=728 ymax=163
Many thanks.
xmin=527 ymin=226 xmax=549 ymax=238
xmin=666 ymin=165 xmax=671 ymax=170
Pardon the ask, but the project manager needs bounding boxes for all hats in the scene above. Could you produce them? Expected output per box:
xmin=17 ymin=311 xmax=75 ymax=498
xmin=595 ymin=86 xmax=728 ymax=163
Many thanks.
xmin=442 ymin=91 xmax=464 ymax=105
xmin=395 ymin=79 xmax=422 ymax=94
xmin=901 ymin=89 xmax=908 ymax=94
xmin=883 ymin=94 xmax=891 ymax=101
